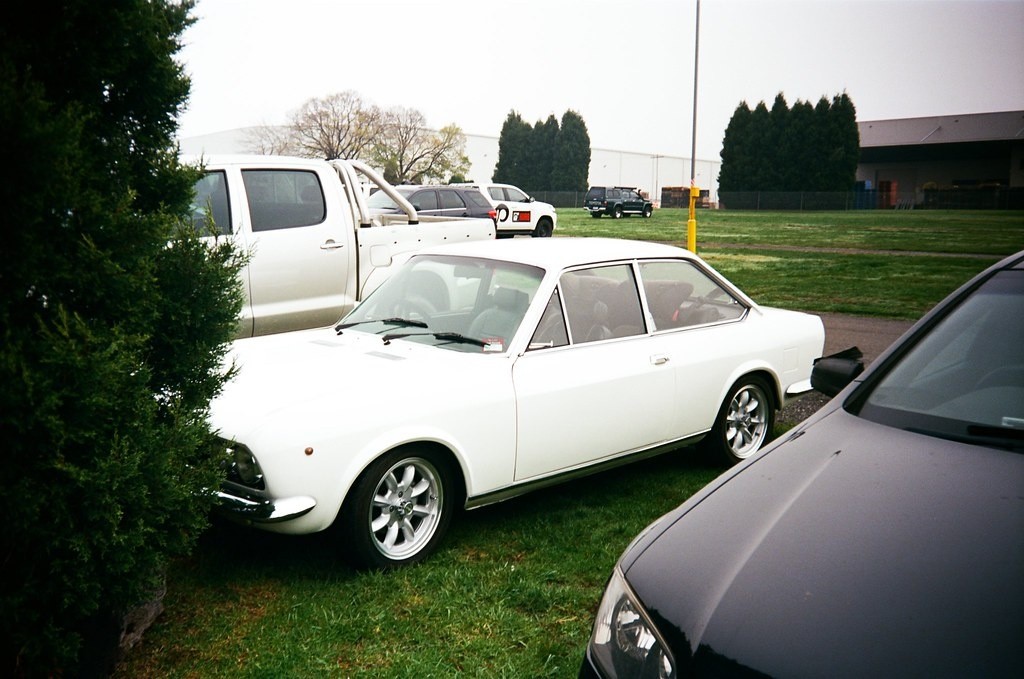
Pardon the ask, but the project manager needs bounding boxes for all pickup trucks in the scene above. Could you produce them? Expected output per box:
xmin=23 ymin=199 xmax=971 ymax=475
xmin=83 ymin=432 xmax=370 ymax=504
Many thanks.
xmin=447 ymin=182 xmax=558 ymax=239
xmin=160 ymin=153 xmax=499 ymax=345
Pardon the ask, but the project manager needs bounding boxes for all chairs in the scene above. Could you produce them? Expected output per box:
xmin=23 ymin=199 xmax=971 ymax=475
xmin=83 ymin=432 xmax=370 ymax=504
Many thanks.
xmin=300 ymin=185 xmax=324 ymax=225
xmin=468 ymin=286 xmax=529 ymax=349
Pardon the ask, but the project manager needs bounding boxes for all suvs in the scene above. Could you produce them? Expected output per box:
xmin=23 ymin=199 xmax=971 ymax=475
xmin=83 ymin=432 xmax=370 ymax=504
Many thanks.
xmin=583 ymin=185 xmax=654 ymax=219
xmin=363 ymin=182 xmax=498 ymax=239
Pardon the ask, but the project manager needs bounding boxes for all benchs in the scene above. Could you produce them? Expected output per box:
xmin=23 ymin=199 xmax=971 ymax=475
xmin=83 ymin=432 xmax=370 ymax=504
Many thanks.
xmin=561 ymin=274 xmax=700 ymax=331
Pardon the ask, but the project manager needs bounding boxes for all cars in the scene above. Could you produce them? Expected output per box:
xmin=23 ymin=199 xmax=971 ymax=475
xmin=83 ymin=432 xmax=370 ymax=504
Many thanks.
xmin=150 ymin=236 xmax=827 ymax=572
xmin=576 ymin=247 xmax=1024 ymax=679
